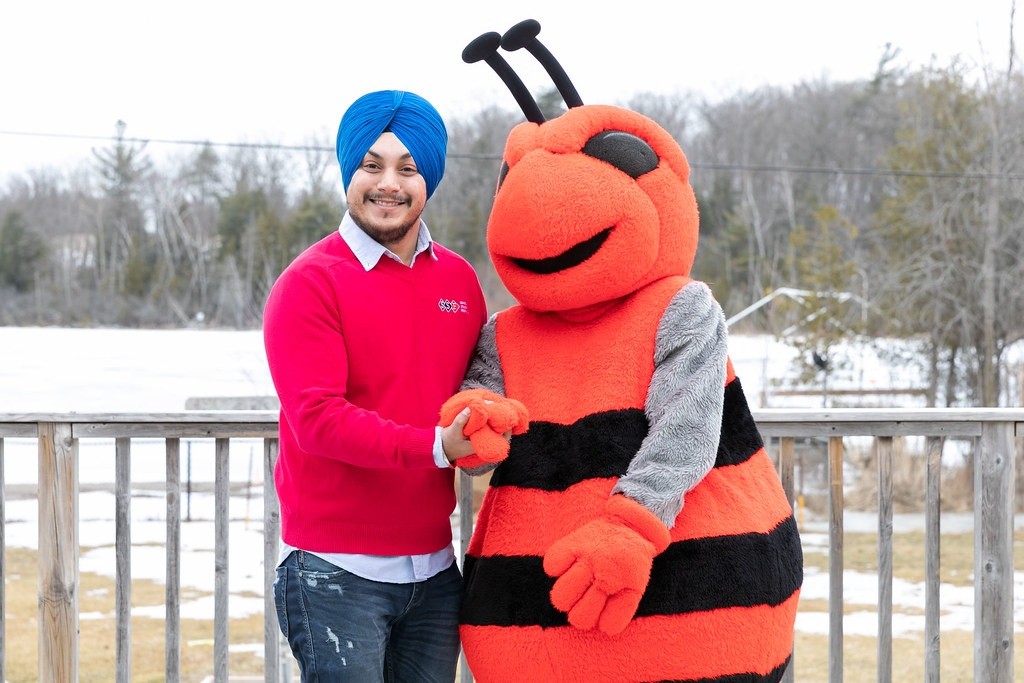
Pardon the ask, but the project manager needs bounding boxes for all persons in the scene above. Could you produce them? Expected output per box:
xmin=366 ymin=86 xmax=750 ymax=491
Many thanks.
xmin=262 ymin=90 xmax=492 ymax=683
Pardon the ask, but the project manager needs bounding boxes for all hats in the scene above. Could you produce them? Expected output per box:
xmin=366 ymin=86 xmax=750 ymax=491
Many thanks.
xmin=337 ymin=91 xmax=448 ymax=201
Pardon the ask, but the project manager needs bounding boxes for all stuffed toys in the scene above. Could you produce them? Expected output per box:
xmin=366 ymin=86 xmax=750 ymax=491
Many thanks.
xmin=436 ymin=20 xmax=805 ymax=683
